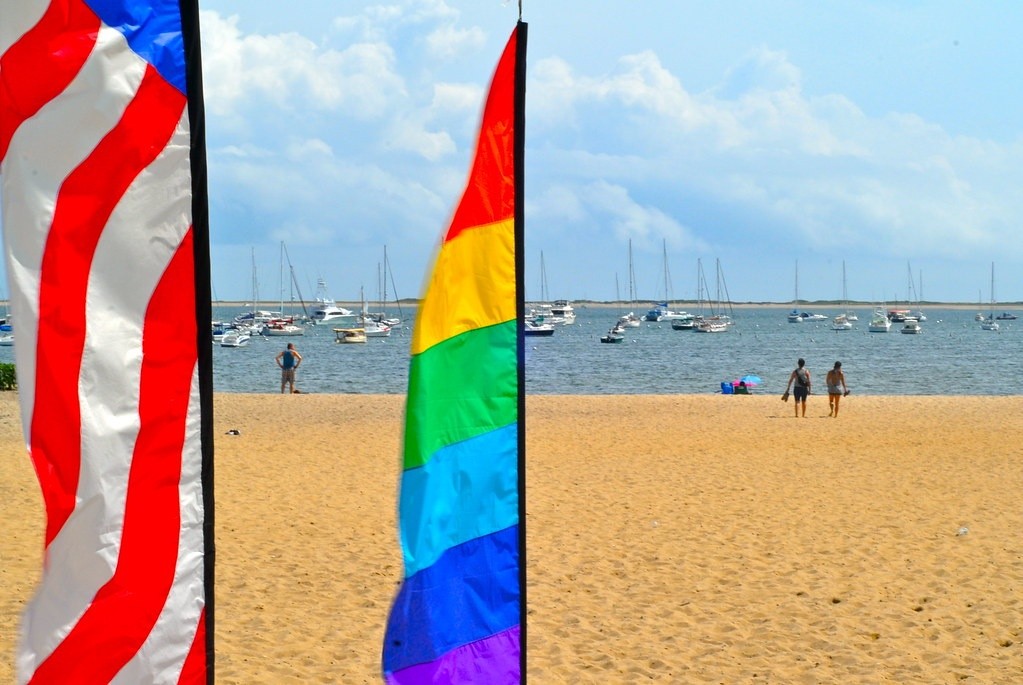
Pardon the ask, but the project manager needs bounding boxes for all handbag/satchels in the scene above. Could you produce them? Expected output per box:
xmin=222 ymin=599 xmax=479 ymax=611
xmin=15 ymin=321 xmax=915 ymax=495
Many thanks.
xmin=796 ymin=370 xmax=806 ymax=387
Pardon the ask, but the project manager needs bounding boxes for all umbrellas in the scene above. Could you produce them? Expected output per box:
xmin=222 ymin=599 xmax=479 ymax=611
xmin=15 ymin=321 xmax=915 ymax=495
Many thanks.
xmin=733 ymin=375 xmax=762 ymax=387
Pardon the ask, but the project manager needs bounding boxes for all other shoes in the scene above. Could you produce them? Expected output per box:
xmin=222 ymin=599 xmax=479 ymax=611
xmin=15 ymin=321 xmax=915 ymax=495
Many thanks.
xmin=844 ymin=390 xmax=850 ymax=397
xmin=781 ymin=393 xmax=789 ymax=402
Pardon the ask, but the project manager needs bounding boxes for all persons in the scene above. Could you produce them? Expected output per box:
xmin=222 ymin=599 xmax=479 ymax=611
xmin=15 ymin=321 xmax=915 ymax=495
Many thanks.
xmin=826 ymin=361 xmax=847 ymax=417
xmin=276 ymin=343 xmax=302 ymax=394
xmin=738 ymin=381 xmax=745 ymax=388
xmin=786 ymin=358 xmax=811 ymax=417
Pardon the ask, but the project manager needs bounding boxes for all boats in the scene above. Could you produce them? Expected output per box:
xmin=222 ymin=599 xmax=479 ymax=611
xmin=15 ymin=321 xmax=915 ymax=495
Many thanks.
xmin=210 ymin=235 xmax=410 ymax=349
xmin=867 ymin=299 xmax=892 ymax=333
xmin=523 ymin=246 xmax=575 ymax=338
xmin=995 ymin=312 xmax=1019 ymax=319
xmin=599 ymin=327 xmax=624 ymax=345
xmin=899 ymin=318 xmax=921 ymax=334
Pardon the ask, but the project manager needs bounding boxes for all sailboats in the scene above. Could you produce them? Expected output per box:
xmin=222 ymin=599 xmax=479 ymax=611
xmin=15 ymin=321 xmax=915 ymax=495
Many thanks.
xmin=606 ymin=237 xmax=736 ymax=336
xmin=784 ymin=257 xmax=859 ymax=332
xmin=974 ymin=287 xmax=984 ymax=322
xmin=887 ymin=259 xmax=927 ymax=322
xmin=981 ymin=261 xmax=999 ymax=330
xmin=0 ymin=296 xmax=15 ymax=347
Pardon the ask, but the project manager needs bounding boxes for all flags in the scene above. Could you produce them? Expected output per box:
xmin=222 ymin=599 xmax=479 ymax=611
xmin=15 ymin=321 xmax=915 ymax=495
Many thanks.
xmin=382 ymin=22 xmax=527 ymax=685
xmin=0 ymin=0 xmax=216 ymax=685
